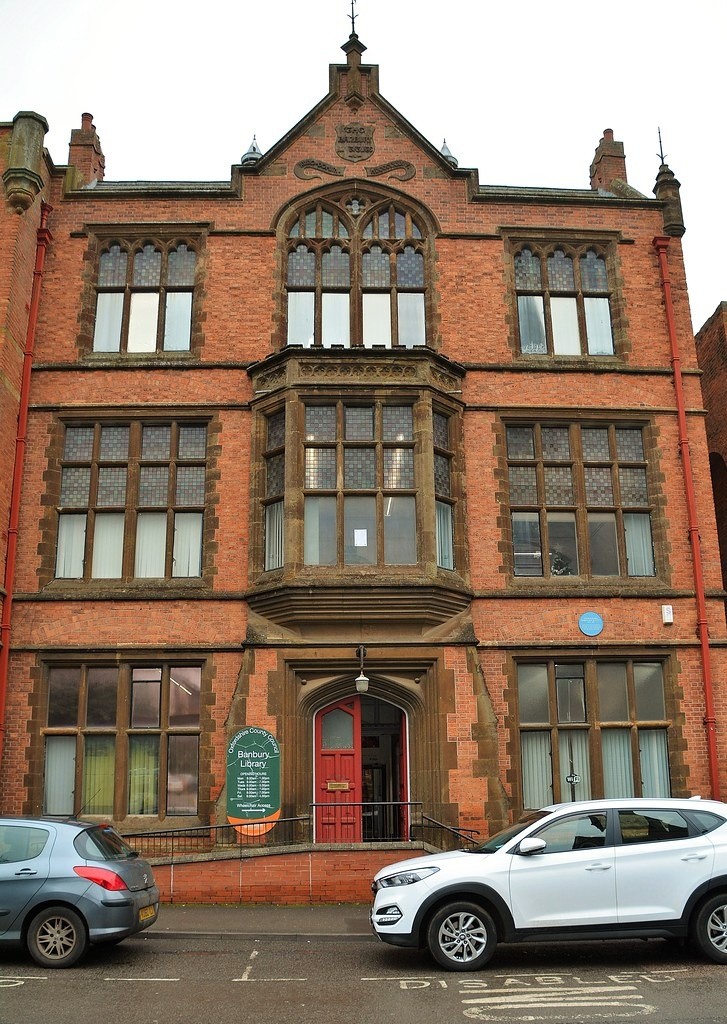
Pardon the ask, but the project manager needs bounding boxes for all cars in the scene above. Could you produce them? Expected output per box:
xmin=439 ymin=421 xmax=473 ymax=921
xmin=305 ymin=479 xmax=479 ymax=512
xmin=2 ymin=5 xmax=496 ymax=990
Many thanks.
xmin=0 ymin=815 xmax=161 ymax=970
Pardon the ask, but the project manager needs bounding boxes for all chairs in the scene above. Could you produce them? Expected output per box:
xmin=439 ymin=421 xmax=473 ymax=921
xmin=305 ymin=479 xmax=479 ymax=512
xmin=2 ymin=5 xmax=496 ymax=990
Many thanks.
xmin=645 ymin=813 xmax=688 ymax=840
xmin=572 ymin=816 xmax=604 ymax=849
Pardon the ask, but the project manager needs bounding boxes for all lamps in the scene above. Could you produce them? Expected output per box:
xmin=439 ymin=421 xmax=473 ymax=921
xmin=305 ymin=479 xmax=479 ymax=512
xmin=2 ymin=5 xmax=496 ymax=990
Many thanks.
xmin=355 ymin=645 xmax=369 ymax=692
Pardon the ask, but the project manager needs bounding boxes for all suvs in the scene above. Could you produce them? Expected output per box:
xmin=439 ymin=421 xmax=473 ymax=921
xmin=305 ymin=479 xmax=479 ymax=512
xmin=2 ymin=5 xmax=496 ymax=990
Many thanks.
xmin=372 ymin=795 xmax=727 ymax=972
